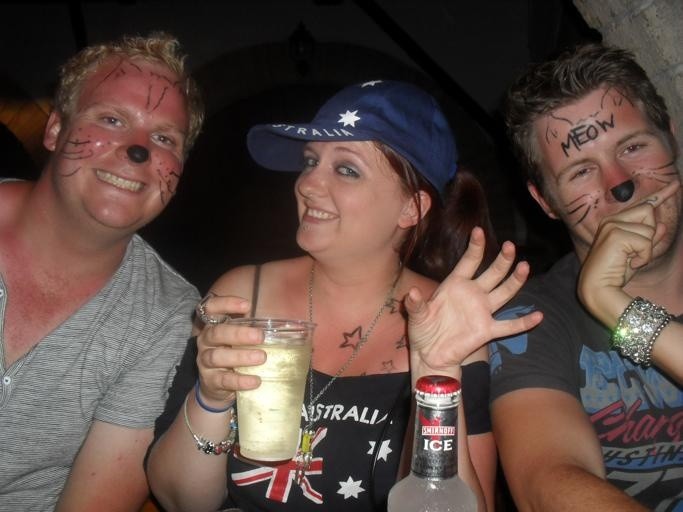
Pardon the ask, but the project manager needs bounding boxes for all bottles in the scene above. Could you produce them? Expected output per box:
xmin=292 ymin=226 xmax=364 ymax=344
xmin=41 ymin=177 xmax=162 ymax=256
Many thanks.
xmin=385 ymin=372 xmax=480 ymax=510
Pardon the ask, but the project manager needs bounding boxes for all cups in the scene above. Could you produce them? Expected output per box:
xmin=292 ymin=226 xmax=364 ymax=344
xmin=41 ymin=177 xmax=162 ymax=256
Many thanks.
xmin=223 ymin=316 xmax=316 ymax=462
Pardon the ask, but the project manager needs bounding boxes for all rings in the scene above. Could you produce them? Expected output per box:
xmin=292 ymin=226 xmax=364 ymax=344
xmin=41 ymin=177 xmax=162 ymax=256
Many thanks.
xmin=193 ymin=292 xmax=228 ymax=324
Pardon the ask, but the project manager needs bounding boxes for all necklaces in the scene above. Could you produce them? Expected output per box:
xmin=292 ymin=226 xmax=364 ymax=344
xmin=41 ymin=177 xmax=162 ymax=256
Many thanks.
xmin=299 ymin=260 xmax=402 ymax=469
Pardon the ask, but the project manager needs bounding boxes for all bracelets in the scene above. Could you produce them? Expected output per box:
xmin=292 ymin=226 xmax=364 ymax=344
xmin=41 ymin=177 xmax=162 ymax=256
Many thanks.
xmin=193 ymin=378 xmax=235 ymax=412
xmin=610 ymin=295 xmax=674 ymax=369
xmin=183 ymin=391 xmax=237 ymax=455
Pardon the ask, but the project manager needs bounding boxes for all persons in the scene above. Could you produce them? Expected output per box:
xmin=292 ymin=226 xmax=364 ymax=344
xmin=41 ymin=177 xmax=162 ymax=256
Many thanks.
xmin=0 ymin=32 xmax=206 ymax=511
xmin=575 ymin=179 xmax=683 ymax=391
xmin=0 ymin=98 xmax=55 ymax=183
xmin=142 ymin=78 xmax=546 ymax=510
xmin=483 ymin=41 xmax=682 ymax=512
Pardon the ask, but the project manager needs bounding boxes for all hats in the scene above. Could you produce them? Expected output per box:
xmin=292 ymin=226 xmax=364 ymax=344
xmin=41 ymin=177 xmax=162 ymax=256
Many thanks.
xmin=246 ymin=79 xmax=459 ymax=193
